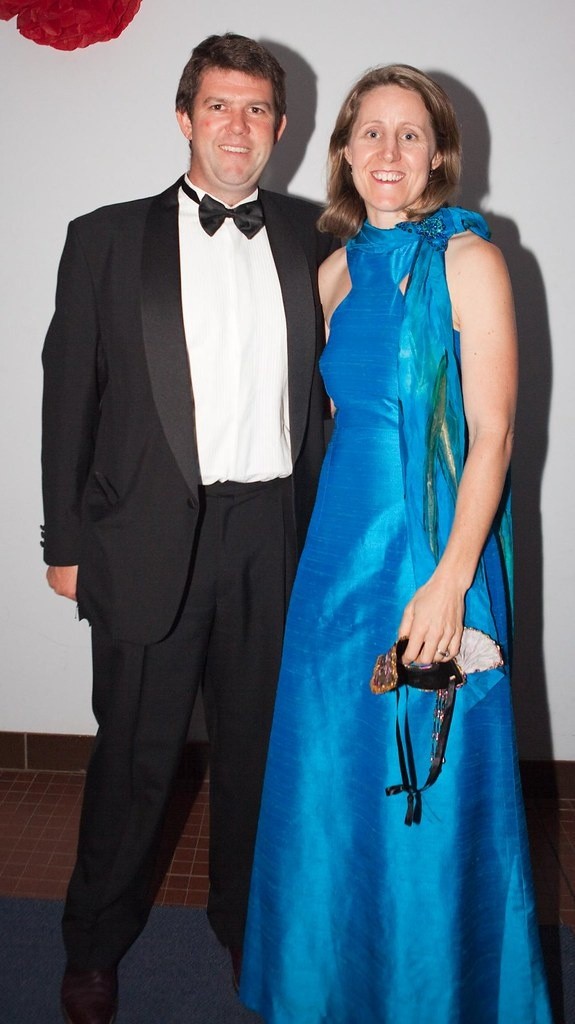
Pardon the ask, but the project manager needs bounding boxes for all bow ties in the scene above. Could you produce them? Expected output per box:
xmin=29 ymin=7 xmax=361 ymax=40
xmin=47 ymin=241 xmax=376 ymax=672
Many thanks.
xmin=198 ymin=195 xmax=267 ymax=240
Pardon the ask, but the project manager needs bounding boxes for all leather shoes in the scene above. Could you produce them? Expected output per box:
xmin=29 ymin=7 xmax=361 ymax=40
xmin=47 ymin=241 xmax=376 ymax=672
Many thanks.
xmin=225 ymin=939 xmax=246 ymax=994
xmin=58 ymin=962 xmax=122 ymax=1023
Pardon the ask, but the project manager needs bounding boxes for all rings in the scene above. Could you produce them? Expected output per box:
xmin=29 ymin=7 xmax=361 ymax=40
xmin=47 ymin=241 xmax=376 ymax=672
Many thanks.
xmin=438 ymin=649 xmax=449 ymax=657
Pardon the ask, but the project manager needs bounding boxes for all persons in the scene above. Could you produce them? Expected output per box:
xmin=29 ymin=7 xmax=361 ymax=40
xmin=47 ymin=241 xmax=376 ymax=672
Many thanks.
xmin=39 ymin=32 xmax=341 ymax=1024
xmin=240 ymin=62 xmax=554 ymax=1024
